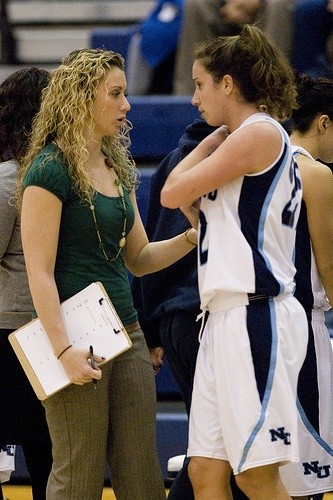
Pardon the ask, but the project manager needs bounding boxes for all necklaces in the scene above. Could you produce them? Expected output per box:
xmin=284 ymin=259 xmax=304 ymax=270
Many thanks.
xmin=88 ymin=179 xmax=127 ymax=262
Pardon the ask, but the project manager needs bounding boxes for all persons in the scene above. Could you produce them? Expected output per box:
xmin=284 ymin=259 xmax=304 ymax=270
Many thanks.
xmin=0 ymin=67 xmax=54 ymax=500
xmin=159 ymin=27 xmax=307 ymax=500
xmin=14 ymin=50 xmax=196 ymax=500
xmin=279 ymin=74 xmax=333 ymax=500
xmin=175 ymin=0 xmax=296 ymax=97
xmin=133 ymin=123 xmax=247 ymax=500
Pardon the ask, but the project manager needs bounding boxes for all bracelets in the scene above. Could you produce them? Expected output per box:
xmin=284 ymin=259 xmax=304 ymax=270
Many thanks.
xmin=57 ymin=345 xmax=72 ymax=359
xmin=185 ymin=228 xmax=196 ymax=246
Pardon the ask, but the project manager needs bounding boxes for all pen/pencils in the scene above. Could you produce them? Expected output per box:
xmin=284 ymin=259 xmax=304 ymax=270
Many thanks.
xmin=90 ymin=346 xmax=97 ymax=390
